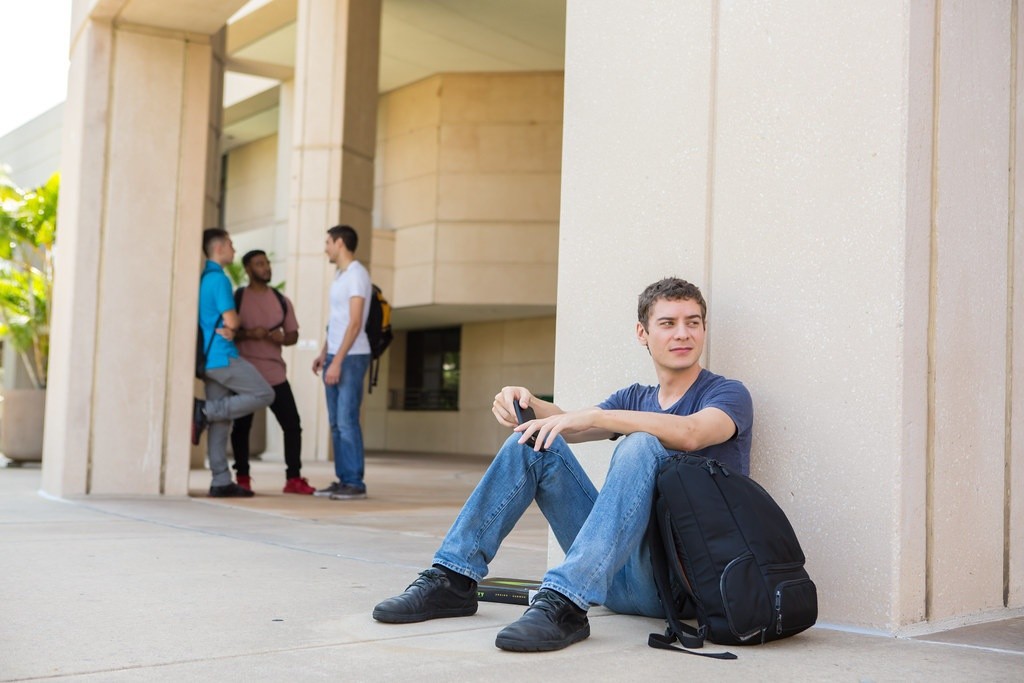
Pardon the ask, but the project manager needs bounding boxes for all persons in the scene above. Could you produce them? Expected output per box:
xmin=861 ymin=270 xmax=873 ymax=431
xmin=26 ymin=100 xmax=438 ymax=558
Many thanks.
xmin=193 ymin=226 xmax=316 ymax=498
xmin=312 ymin=225 xmax=373 ymax=500
xmin=372 ymin=278 xmax=755 ymax=651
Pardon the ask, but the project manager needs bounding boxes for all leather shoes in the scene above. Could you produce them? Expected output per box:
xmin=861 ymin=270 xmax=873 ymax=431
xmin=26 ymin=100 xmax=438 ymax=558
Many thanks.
xmin=210 ymin=484 xmax=254 ymax=497
xmin=373 ymin=567 xmax=479 ymax=623
xmin=192 ymin=397 xmax=207 ymax=446
xmin=495 ymin=590 xmax=591 ymax=651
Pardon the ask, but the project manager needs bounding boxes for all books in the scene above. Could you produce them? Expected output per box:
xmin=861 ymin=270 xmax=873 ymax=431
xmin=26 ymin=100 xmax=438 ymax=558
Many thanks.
xmin=477 ymin=576 xmax=544 ymax=606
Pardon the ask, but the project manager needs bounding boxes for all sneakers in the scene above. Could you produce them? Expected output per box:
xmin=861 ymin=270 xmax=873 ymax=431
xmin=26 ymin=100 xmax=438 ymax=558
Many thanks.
xmin=314 ymin=483 xmax=343 ymax=497
xmin=284 ymin=477 xmax=317 ymax=494
xmin=234 ymin=476 xmax=252 ymax=491
xmin=329 ymin=486 xmax=368 ymax=499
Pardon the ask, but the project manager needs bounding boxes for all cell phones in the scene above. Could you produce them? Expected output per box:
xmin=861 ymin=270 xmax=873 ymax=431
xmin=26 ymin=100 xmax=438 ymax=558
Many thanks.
xmin=513 ymin=400 xmax=546 ymax=453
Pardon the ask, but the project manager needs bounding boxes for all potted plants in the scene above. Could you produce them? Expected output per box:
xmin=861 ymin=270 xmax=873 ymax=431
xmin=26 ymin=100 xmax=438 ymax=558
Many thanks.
xmin=0 ymin=172 xmax=62 ymax=461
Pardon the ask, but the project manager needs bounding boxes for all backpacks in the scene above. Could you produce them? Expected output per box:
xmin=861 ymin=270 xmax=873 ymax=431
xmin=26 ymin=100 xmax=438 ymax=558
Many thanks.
xmin=195 ymin=309 xmax=225 ymax=380
xmin=648 ymin=451 xmax=819 ymax=659
xmin=365 ymin=284 xmax=395 ymax=358
